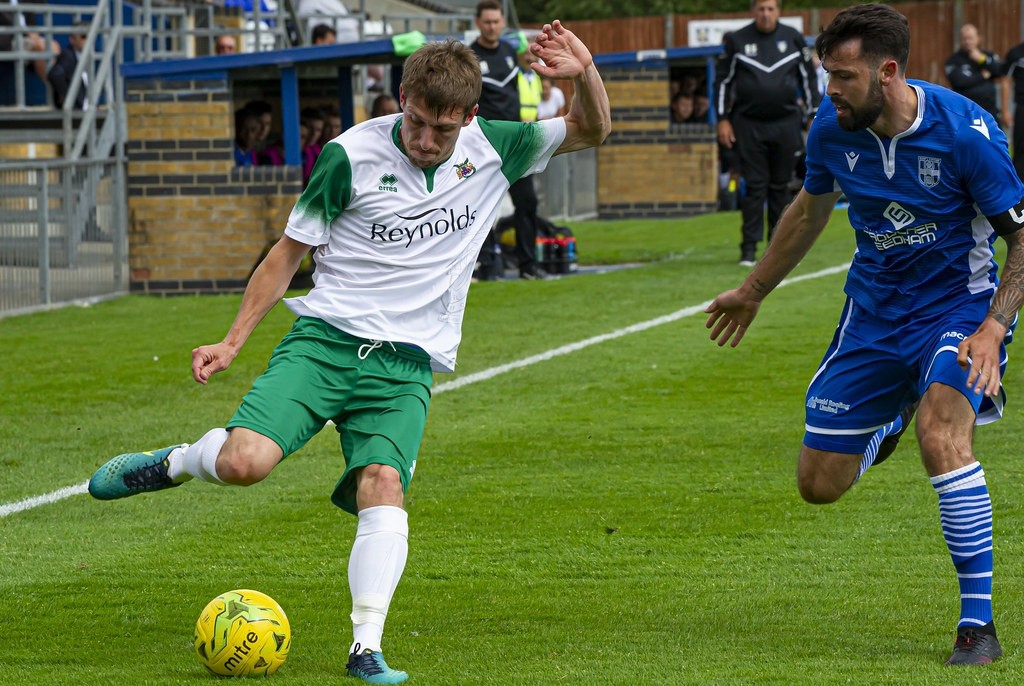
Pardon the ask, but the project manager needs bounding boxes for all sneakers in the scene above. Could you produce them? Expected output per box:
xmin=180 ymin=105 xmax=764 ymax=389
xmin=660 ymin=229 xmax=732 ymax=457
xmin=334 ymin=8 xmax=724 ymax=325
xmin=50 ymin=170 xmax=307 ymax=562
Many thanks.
xmin=345 ymin=647 xmax=408 ymax=684
xmin=519 ymin=265 xmax=552 ymax=281
xmin=738 ymin=242 xmax=757 ymax=267
xmin=88 ymin=443 xmax=195 ymax=501
xmin=870 ymin=389 xmax=921 ymax=465
xmin=943 ymin=619 xmax=1003 ymax=667
xmin=472 ymin=267 xmax=503 ymax=282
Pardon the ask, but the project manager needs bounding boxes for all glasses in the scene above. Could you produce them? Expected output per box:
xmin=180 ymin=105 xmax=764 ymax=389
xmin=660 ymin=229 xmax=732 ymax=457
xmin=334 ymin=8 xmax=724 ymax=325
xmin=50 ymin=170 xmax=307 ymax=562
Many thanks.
xmin=81 ymin=35 xmax=85 ymax=38
xmin=219 ymin=45 xmax=234 ymax=50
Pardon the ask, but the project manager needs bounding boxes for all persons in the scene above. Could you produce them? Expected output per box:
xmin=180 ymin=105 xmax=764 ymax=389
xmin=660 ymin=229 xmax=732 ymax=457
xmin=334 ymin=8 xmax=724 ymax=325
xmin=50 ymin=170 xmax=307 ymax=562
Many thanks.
xmin=668 ymin=30 xmax=846 ymax=266
xmin=214 ymin=1 xmax=567 ymax=283
xmin=705 ymin=3 xmax=1024 ymax=668
xmin=0 ymin=7 xmax=112 ymax=158
xmin=942 ymin=24 xmax=1024 ymax=185
xmin=88 ymin=19 xmax=613 ymax=686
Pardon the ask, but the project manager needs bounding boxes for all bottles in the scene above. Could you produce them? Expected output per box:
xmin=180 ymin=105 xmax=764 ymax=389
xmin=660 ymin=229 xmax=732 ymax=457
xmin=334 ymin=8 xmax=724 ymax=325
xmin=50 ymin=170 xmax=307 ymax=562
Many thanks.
xmin=535 ymin=237 xmax=578 ymax=273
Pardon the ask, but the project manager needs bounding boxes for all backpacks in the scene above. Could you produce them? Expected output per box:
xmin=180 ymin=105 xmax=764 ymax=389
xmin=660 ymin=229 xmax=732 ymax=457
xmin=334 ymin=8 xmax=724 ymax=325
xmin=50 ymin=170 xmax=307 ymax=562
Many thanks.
xmin=496 ymin=215 xmax=581 ymax=279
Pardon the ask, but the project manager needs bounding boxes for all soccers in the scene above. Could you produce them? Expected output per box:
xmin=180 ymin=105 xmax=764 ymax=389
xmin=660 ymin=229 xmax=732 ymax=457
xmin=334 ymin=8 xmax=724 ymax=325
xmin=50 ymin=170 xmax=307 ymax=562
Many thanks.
xmin=194 ymin=589 xmax=291 ymax=677
